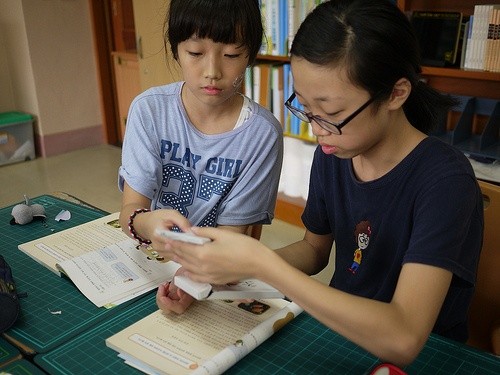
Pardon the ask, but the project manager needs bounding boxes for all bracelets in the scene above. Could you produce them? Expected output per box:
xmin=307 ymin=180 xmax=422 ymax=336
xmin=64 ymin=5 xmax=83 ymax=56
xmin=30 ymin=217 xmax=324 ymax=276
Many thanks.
xmin=128 ymin=208 xmax=152 ymax=244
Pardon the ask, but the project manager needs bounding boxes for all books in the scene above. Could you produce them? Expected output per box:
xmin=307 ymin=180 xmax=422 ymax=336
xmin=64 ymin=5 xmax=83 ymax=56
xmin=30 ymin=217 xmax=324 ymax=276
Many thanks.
xmin=18 ymin=211 xmax=183 ymax=309
xmin=244 ymin=63 xmax=316 ymax=139
xmin=104 ymin=298 xmax=304 ymax=375
xmin=461 ymin=5 xmax=500 ymax=73
xmin=257 ymin=0 xmax=325 ymax=57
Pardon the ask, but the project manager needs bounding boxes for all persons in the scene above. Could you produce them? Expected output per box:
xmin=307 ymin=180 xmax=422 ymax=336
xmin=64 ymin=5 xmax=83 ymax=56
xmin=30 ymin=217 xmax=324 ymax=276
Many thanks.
xmin=116 ymin=0 xmax=283 ymax=315
xmin=164 ymin=0 xmax=484 ymax=368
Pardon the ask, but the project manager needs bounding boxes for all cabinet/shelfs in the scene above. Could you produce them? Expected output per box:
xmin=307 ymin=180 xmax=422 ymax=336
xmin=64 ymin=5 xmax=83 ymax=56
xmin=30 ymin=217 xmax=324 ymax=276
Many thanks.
xmin=240 ymin=0 xmax=500 ymax=181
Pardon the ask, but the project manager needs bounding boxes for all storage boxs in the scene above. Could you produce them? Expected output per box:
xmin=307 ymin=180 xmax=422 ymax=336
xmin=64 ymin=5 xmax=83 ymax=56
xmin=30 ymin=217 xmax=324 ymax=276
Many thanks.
xmin=0 ymin=111 xmax=37 ymax=167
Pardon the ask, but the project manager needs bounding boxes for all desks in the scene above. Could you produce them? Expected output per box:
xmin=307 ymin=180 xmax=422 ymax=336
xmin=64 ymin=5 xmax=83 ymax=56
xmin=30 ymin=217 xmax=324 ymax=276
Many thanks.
xmin=0 ymin=191 xmax=500 ymax=375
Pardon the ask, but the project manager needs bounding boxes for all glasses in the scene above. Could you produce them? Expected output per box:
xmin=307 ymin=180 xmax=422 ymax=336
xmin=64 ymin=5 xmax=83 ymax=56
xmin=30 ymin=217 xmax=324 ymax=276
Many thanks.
xmin=285 ymin=89 xmax=384 ymax=135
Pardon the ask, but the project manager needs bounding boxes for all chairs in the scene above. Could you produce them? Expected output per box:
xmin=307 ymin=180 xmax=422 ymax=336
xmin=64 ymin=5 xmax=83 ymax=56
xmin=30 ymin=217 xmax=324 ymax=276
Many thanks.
xmin=465 ymin=180 xmax=500 ymax=357
